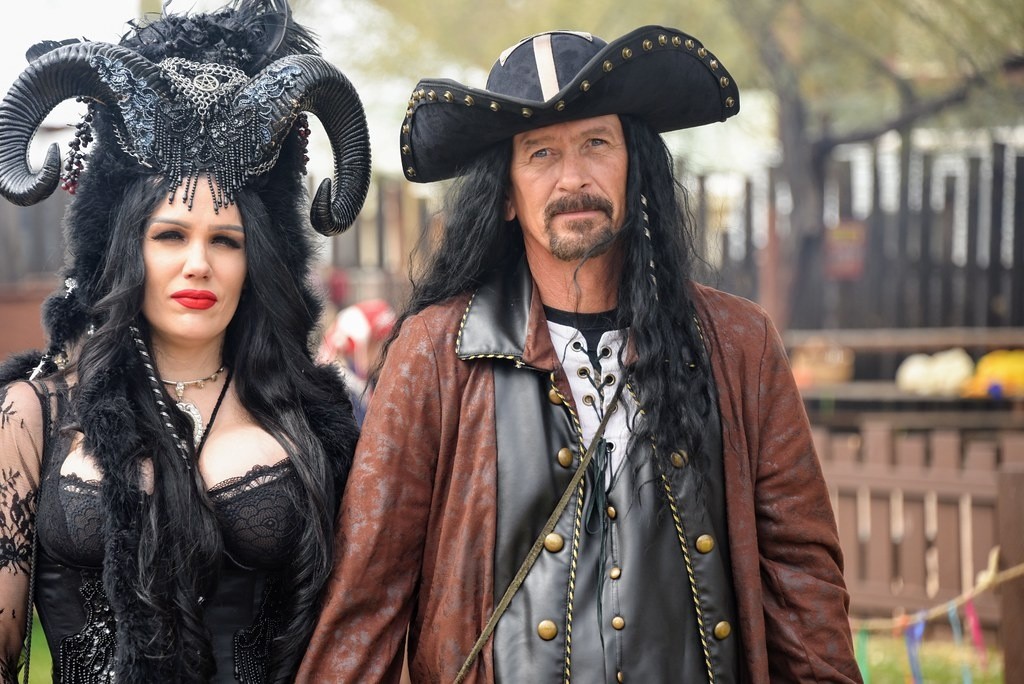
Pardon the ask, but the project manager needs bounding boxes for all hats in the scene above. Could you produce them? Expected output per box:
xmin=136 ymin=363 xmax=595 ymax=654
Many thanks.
xmin=399 ymin=24 xmax=740 ymax=183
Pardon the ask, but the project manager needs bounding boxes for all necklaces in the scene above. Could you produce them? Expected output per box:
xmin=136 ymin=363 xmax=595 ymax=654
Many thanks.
xmin=120 ymin=350 xmax=239 ymax=454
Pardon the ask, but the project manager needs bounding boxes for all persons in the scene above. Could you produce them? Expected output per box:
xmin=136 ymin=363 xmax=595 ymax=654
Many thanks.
xmin=290 ymin=28 xmax=850 ymax=684
xmin=1 ymin=105 xmax=375 ymax=684
